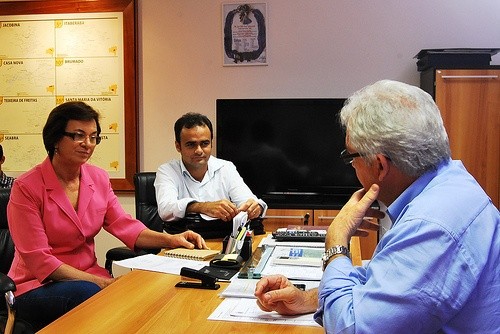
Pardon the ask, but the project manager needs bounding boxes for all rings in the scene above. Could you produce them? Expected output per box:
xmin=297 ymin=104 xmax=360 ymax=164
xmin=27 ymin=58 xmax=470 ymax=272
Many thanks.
xmin=253 ymin=211 xmax=256 ymax=215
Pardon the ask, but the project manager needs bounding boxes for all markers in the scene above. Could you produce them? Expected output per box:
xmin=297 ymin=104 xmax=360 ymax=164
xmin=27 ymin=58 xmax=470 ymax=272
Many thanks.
xmin=225 ymin=235 xmax=234 ymax=254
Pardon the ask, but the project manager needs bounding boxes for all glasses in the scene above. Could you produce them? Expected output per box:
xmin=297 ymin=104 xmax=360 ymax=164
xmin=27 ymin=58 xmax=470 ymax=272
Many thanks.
xmin=339 ymin=147 xmax=392 ymax=164
xmin=63 ymin=131 xmax=101 ymax=146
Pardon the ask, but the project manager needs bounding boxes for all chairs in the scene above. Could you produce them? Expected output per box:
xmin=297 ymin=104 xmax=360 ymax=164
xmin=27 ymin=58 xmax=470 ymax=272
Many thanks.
xmin=0 ymin=187 xmax=33 ymax=334
xmin=101 ymin=168 xmax=167 ymax=273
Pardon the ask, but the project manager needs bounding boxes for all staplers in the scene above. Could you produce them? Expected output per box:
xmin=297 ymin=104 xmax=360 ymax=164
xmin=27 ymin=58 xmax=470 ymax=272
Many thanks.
xmin=175 ymin=267 xmax=220 ymax=290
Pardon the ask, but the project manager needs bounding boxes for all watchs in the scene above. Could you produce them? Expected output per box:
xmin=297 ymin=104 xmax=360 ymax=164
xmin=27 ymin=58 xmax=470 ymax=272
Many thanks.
xmin=323 ymin=245 xmax=352 ymax=271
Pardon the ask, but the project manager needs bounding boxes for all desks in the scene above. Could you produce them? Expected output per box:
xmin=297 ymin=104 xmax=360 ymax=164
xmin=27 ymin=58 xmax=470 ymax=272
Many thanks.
xmin=33 ymin=231 xmax=362 ymax=334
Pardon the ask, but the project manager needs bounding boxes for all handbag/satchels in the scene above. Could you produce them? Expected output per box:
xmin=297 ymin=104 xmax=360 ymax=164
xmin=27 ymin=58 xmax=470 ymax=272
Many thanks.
xmin=163 ymin=213 xmax=265 ymax=239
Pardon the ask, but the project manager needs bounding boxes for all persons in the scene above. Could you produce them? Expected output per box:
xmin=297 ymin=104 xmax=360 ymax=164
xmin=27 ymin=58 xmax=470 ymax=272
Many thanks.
xmin=153 ymin=112 xmax=268 ymax=240
xmin=7 ymin=102 xmax=210 ymax=328
xmin=254 ymin=79 xmax=500 ymax=334
xmin=0 ymin=145 xmax=17 ymax=188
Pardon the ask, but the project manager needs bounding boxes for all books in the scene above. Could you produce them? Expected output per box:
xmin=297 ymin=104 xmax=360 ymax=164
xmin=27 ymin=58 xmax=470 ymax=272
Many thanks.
xmin=165 ymin=247 xmax=221 ymax=261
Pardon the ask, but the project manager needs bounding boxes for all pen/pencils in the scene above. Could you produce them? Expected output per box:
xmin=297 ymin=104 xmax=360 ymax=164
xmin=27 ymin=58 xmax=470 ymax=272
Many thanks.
xmin=235 ymin=219 xmax=251 ymax=241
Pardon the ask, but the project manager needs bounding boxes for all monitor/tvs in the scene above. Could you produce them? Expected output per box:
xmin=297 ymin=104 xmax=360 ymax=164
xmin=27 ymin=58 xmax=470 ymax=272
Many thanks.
xmin=216 ymin=98 xmax=364 ymax=204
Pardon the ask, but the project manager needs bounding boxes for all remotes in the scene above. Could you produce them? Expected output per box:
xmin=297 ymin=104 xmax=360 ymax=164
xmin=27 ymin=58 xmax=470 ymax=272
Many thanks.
xmin=272 ymin=230 xmax=320 ymax=238
xmin=275 ymin=234 xmax=326 ymax=242
